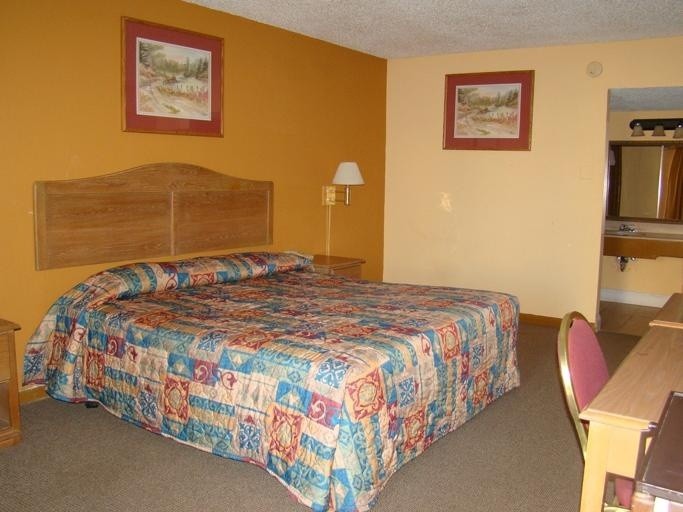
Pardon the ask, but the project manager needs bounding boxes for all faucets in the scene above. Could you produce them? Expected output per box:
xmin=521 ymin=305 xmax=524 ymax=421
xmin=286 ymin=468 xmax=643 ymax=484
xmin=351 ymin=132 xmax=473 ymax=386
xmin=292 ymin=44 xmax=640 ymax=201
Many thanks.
xmin=619 ymin=223 xmax=641 ymax=232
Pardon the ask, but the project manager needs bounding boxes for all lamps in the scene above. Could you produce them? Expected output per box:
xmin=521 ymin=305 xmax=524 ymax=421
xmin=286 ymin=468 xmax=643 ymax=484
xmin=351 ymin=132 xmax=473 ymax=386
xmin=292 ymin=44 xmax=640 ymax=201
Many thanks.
xmin=321 ymin=162 xmax=365 ymax=207
xmin=630 ymin=118 xmax=683 ymax=138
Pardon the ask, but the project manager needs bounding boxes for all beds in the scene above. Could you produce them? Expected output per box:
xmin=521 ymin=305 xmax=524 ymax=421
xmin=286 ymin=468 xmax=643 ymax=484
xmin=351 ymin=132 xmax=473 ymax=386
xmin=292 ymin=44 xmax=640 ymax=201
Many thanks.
xmin=23 ymin=269 xmax=521 ymax=512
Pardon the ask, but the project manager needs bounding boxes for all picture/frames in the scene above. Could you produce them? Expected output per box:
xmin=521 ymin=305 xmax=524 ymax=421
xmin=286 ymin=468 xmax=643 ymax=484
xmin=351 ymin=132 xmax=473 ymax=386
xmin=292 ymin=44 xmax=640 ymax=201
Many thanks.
xmin=120 ymin=16 xmax=225 ymax=138
xmin=442 ymin=71 xmax=534 ymax=151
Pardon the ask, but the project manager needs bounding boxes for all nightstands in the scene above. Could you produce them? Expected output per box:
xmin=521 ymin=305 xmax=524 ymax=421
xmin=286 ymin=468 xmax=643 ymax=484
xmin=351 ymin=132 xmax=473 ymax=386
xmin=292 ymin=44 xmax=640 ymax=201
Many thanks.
xmin=312 ymin=255 xmax=366 ymax=280
xmin=0 ymin=319 xmax=21 ymax=446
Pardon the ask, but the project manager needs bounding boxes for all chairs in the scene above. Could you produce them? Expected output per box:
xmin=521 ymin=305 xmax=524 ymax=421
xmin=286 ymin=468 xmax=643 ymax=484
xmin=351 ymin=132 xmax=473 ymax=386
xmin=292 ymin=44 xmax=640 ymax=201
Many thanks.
xmin=557 ymin=312 xmax=660 ymax=507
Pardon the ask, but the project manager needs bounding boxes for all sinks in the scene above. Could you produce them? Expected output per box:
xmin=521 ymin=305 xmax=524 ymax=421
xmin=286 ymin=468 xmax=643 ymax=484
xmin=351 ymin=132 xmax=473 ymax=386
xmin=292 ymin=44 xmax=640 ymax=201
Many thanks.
xmin=606 ymin=230 xmax=642 ymax=236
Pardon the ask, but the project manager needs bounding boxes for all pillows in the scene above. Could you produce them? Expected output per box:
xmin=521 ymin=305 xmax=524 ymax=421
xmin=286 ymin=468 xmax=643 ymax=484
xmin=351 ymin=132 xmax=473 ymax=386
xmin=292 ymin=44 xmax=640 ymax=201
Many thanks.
xmin=86 ymin=245 xmax=312 ymax=299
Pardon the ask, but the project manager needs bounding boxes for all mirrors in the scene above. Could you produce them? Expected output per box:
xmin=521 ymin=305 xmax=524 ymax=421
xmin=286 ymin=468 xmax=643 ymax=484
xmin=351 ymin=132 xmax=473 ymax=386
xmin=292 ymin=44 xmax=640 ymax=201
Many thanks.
xmin=605 ymin=141 xmax=683 ymax=225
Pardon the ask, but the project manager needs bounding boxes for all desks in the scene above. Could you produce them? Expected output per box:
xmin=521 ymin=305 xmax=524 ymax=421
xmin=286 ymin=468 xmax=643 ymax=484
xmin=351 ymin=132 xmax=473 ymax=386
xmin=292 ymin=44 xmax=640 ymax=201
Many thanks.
xmin=579 ymin=326 xmax=683 ymax=512
xmin=648 ymin=293 xmax=683 ymax=329
